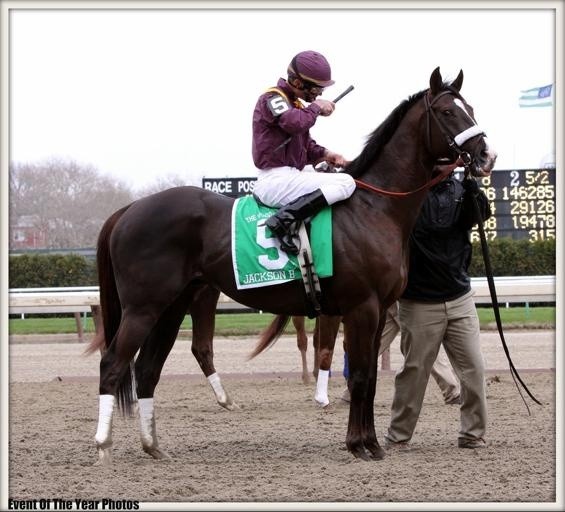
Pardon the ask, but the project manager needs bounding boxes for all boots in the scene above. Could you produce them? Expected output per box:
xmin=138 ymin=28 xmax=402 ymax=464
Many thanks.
xmin=265 ymin=188 xmax=329 ymax=256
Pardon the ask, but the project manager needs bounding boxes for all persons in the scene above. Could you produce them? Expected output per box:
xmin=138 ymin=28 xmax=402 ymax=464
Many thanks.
xmin=340 ymin=157 xmax=491 ymax=449
xmin=252 ymin=51 xmax=357 ymax=255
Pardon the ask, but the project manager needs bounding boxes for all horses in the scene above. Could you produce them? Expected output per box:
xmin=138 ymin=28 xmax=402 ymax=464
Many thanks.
xmin=76 ymin=67 xmax=498 ymax=468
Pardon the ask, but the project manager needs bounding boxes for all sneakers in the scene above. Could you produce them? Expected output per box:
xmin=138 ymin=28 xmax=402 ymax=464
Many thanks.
xmin=459 ymin=436 xmax=486 ymax=448
xmin=377 ymin=438 xmax=409 ymax=451
xmin=446 ymin=392 xmax=462 ymax=405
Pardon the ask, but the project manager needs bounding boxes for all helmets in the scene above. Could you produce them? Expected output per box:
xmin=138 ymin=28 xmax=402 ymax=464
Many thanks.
xmin=287 ymin=49 xmax=336 ymax=88
